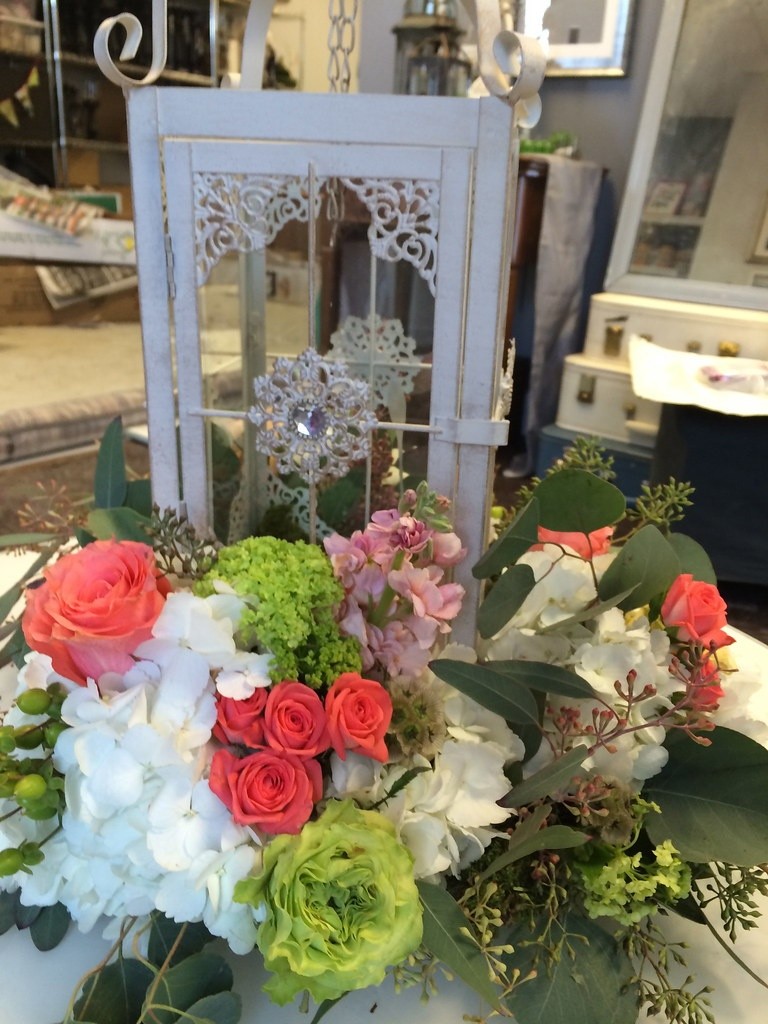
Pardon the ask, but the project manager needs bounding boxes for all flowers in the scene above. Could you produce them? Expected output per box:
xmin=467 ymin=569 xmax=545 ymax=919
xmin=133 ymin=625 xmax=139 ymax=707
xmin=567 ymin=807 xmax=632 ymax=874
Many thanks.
xmin=0 ymin=412 xmax=768 ymax=1024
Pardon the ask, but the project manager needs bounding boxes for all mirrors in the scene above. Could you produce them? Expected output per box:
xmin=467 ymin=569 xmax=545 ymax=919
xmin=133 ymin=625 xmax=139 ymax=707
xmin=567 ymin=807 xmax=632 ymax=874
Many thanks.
xmin=602 ymin=0 xmax=768 ymax=313
